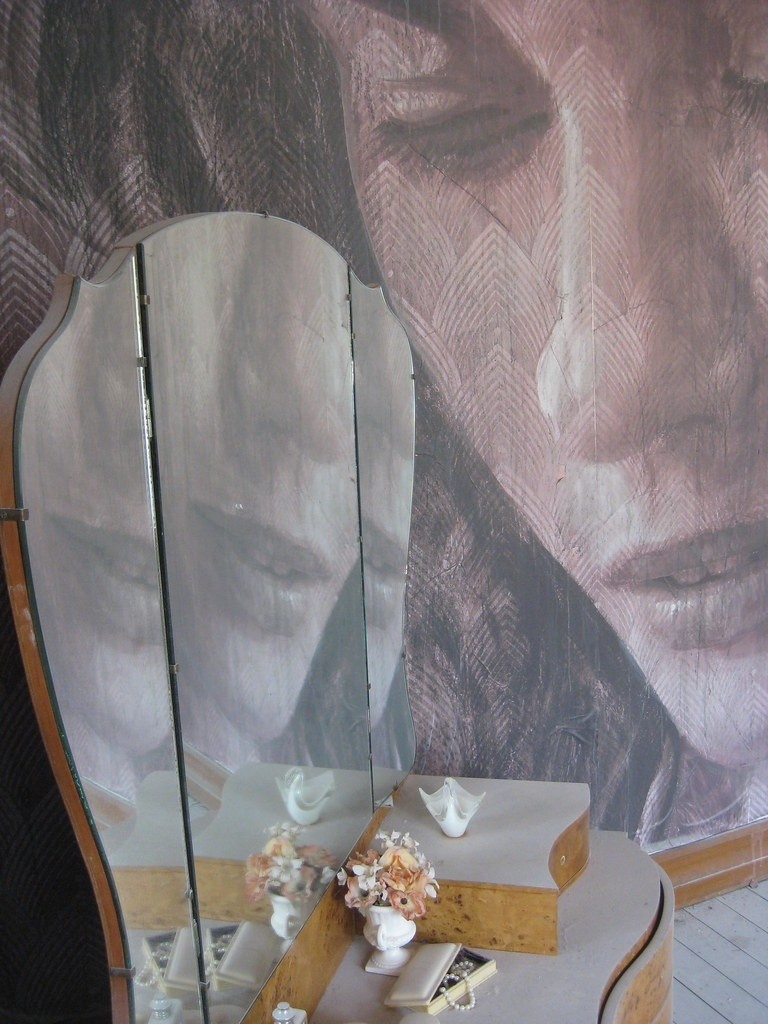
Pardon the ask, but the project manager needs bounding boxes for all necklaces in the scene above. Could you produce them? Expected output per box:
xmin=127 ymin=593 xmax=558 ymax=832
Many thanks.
xmin=440 ymin=960 xmax=476 ymax=1010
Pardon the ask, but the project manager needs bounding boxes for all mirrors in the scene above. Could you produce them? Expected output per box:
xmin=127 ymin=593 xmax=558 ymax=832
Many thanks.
xmin=18 ymin=193 xmax=432 ymax=1024
